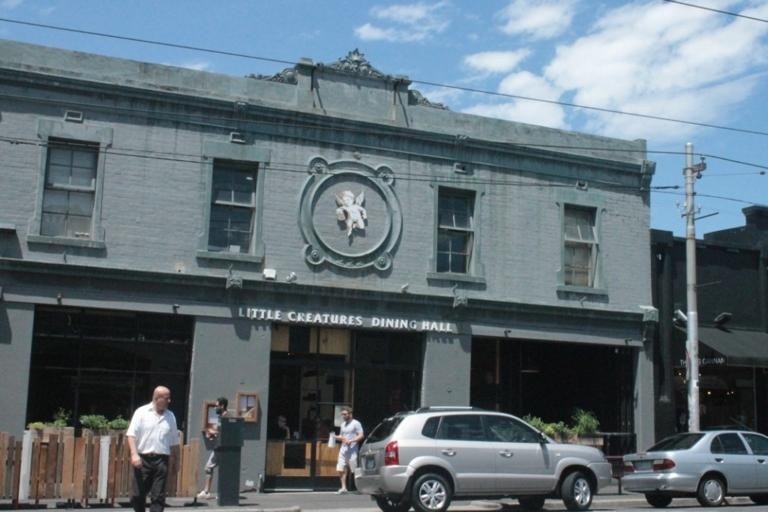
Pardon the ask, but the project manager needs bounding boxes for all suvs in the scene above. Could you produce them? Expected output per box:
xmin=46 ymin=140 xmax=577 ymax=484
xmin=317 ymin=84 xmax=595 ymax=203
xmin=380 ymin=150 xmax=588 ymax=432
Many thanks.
xmin=351 ymin=403 xmax=612 ymax=510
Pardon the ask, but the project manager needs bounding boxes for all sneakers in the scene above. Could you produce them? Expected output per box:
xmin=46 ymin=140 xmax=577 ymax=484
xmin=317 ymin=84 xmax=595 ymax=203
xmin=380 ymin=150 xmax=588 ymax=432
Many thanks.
xmin=337 ymin=489 xmax=348 ymax=495
xmin=197 ymin=491 xmax=210 ymax=498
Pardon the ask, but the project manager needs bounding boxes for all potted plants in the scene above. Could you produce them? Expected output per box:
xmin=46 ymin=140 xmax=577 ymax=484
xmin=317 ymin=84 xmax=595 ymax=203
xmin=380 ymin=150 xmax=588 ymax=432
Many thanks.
xmin=79 ymin=414 xmax=108 ymax=441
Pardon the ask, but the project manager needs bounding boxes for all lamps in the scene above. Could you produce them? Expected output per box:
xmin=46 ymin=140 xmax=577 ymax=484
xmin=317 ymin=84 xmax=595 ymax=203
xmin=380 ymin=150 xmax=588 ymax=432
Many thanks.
xmin=224 ymin=262 xmax=243 ymax=292
xmin=451 ymin=282 xmax=469 ymax=309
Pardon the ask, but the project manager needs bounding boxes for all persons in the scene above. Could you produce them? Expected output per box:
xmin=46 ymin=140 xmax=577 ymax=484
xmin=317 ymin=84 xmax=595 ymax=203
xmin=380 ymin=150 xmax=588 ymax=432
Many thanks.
xmin=330 ymin=406 xmax=365 ymax=495
xmin=194 ymin=397 xmax=237 ymax=500
xmin=125 ymin=383 xmax=181 ymax=512
xmin=312 ymin=415 xmax=330 ymax=438
xmin=297 ymin=407 xmax=320 ymax=453
xmin=333 ymin=187 xmax=369 ymax=237
xmin=270 ymin=413 xmax=291 ymax=439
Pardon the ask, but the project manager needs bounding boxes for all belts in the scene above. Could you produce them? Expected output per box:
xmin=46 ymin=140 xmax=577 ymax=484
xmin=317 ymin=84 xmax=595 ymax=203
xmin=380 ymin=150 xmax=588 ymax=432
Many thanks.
xmin=139 ymin=453 xmax=168 ymax=458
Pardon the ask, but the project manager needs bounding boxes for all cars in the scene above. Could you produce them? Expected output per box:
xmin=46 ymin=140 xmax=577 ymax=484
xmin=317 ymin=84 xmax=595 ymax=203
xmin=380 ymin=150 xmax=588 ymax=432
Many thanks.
xmin=621 ymin=429 xmax=768 ymax=506
